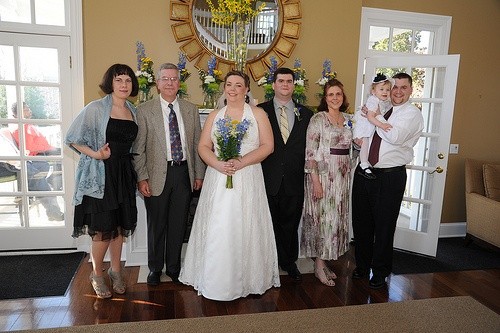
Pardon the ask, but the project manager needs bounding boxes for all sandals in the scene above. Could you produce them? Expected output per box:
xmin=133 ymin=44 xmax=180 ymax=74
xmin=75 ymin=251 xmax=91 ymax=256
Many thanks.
xmin=313 ymin=261 xmax=337 ymax=286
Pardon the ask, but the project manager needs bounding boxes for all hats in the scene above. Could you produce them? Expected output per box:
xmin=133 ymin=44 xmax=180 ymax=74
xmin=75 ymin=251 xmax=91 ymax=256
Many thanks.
xmin=371 ymin=72 xmax=396 ymax=90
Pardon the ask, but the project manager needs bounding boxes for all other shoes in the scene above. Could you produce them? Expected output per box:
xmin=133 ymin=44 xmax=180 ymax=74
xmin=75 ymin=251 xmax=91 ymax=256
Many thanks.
xmin=48 ymin=212 xmax=64 ymax=221
xmin=357 ymin=162 xmax=377 ymax=180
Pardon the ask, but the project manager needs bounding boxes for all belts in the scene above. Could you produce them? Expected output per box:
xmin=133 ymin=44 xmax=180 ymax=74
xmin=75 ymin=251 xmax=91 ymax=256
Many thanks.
xmin=372 ymin=164 xmax=406 ymax=173
xmin=166 ymin=160 xmax=188 ymax=167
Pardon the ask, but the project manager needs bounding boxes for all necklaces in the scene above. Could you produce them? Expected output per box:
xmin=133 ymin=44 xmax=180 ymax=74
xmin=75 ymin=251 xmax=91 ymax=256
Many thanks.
xmin=328 ymin=113 xmax=340 ymax=125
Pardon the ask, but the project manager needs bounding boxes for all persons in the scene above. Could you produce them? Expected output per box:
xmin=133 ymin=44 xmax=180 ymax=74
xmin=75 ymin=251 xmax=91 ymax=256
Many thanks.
xmin=66 ymin=63 xmax=139 ymax=299
xmin=300 ymin=79 xmax=352 ymax=286
xmin=0 ymin=100 xmax=65 ymax=221
xmin=349 ymin=73 xmax=424 ymax=288
xmin=352 ymin=73 xmax=392 ymax=173
xmin=257 ymin=68 xmax=314 ymax=277
xmin=135 ymin=63 xmax=206 ymax=284
xmin=177 ymin=73 xmax=280 ymax=301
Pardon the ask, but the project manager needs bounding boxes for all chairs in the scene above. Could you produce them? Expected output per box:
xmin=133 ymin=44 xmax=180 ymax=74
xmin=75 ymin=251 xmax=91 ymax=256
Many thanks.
xmin=0 ymin=130 xmax=61 ymax=227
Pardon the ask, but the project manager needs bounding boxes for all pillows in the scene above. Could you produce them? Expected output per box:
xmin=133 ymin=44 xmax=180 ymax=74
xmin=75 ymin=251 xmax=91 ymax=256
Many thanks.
xmin=482 ymin=164 xmax=500 ymax=201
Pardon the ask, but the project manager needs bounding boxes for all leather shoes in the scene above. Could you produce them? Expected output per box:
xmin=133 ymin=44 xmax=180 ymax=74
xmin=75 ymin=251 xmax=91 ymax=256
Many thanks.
xmin=147 ymin=270 xmax=162 ymax=285
xmin=164 ymin=270 xmax=183 ymax=284
xmin=285 ymin=262 xmax=303 ymax=280
xmin=368 ymin=274 xmax=385 ymax=288
xmin=352 ymin=265 xmax=371 ymax=278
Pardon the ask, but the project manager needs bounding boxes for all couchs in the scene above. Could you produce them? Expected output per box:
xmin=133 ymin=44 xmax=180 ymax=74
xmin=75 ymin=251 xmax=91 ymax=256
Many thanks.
xmin=464 ymin=159 xmax=500 ymax=249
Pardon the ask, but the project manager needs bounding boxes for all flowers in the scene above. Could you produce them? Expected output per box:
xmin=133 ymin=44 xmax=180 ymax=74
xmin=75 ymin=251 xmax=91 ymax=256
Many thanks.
xmin=178 ymin=52 xmax=192 ymax=95
xmin=290 ymin=58 xmax=309 ymax=106
xmin=294 ymin=107 xmax=303 ymax=121
xmin=262 ymin=56 xmax=279 ymax=101
xmin=344 ymin=118 xmax=354 ymax=129
xmin=212 ymin=114 xmax=253 ymax=189
xmin=206 ymin=0 xmax=265 ymax=28
xmin=199 ymin=55 xmax=223 ymax=93
xmin=315 ymin=57 xmax=337 ymax=102
xmin=136 ymin=41 xmax=155 ymax=91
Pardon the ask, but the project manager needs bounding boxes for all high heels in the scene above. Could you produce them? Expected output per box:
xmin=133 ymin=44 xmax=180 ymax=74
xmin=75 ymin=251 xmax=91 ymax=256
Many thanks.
xmin=107 ymin=266 xmax=127 ymax=294
xmin=88 ymin=271 xmax=112 ymax=298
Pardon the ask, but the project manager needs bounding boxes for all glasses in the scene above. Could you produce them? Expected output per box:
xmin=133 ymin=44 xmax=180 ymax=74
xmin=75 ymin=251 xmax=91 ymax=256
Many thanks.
xmin=160 ymin=78 xmax=179 ymax=82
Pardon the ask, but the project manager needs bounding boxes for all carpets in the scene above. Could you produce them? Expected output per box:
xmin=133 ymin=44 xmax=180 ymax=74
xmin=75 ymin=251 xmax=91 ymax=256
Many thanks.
xmin=0 ymin=294 xmax=500 ymax=333
xmin=0 ymin=251 xmax=86 ymax=299
xmin=392 ymin=237 xmax=500 ymax=273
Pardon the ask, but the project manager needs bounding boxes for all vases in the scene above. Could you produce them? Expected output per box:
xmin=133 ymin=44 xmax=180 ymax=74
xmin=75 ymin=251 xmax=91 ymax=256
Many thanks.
xmin=204 ymin=92 xmax=219 ymax=108
xmin=136 ymin=88 xmax=153 ymax=106
xmin=178 ymin=94 xmax=191 ymax=100
xmin=232 ymin=20 xmax=251 ymax=71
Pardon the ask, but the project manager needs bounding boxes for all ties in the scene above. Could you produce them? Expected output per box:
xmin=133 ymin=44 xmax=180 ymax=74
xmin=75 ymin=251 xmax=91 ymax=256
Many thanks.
xmin=278 ymin=106 xmax=289 ymax=144
xmin=168 ymin=104 xmax=184 ymax=166
xmin=368 ymin=107 xmax=394 ymax=167
